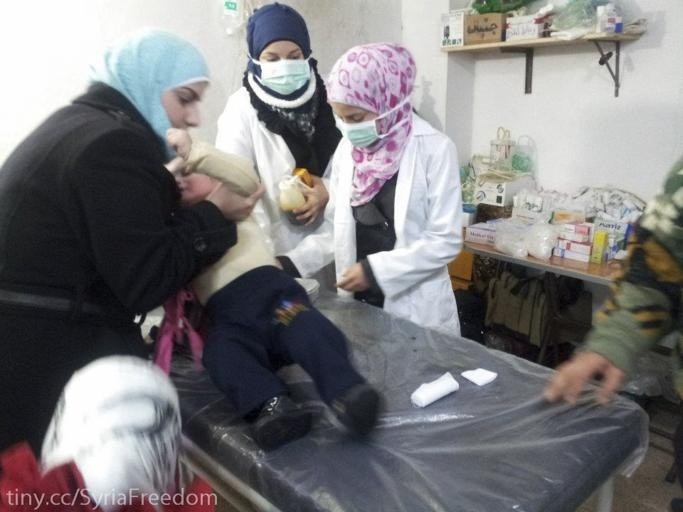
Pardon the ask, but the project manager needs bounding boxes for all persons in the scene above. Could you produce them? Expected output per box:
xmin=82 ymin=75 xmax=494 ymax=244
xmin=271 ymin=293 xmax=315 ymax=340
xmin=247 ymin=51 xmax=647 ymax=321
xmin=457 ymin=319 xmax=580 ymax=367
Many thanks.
xmin=216 ymin=4 xmax=337 ymax=292
xmin=0 ymin=355 xmax=217 ymax=508
xmin=542 ymin=155 xmax=683 ymax=511
xmin=0 ymin=30 xmax=268 ymax=453
xmin=274 ymin=41 xmax=463 ymax=340
xmin=169 ymin=128 xmax=384 ymax=450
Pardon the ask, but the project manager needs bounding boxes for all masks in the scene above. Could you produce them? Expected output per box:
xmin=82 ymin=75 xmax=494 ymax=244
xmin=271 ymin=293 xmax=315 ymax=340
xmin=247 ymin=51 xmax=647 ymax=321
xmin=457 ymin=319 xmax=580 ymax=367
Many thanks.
xmin=333 ymin=91 xmax=412 ymax=148
xmin=245 ymin=51 xmax=314 ymax=95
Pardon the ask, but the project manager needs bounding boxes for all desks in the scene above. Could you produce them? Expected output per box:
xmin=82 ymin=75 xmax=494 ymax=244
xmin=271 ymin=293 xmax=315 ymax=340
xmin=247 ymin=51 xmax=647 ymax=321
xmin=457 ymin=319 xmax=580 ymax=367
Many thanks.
xmin=464 ymin=218 xmax=629 ymax=364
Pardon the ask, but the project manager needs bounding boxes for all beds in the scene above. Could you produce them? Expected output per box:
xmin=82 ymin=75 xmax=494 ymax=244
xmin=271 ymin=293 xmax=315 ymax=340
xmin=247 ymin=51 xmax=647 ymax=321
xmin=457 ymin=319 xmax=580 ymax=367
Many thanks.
xmin=128 ymin=277 xmax=651 ymax=511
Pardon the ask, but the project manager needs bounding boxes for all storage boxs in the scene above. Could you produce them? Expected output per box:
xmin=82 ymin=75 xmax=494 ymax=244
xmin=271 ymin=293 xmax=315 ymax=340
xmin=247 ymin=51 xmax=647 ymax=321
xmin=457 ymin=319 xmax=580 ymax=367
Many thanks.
xmin=465 ymin=13 xmax=507 ymax=44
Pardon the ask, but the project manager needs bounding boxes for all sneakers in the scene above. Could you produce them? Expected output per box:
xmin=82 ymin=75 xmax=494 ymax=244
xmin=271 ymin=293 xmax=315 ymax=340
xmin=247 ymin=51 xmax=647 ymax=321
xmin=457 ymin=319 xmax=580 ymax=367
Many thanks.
xmin=253 ymin=396 xmax=312 ymax=451
xmin=331 ymin=383 xmax=378 ymax=434
